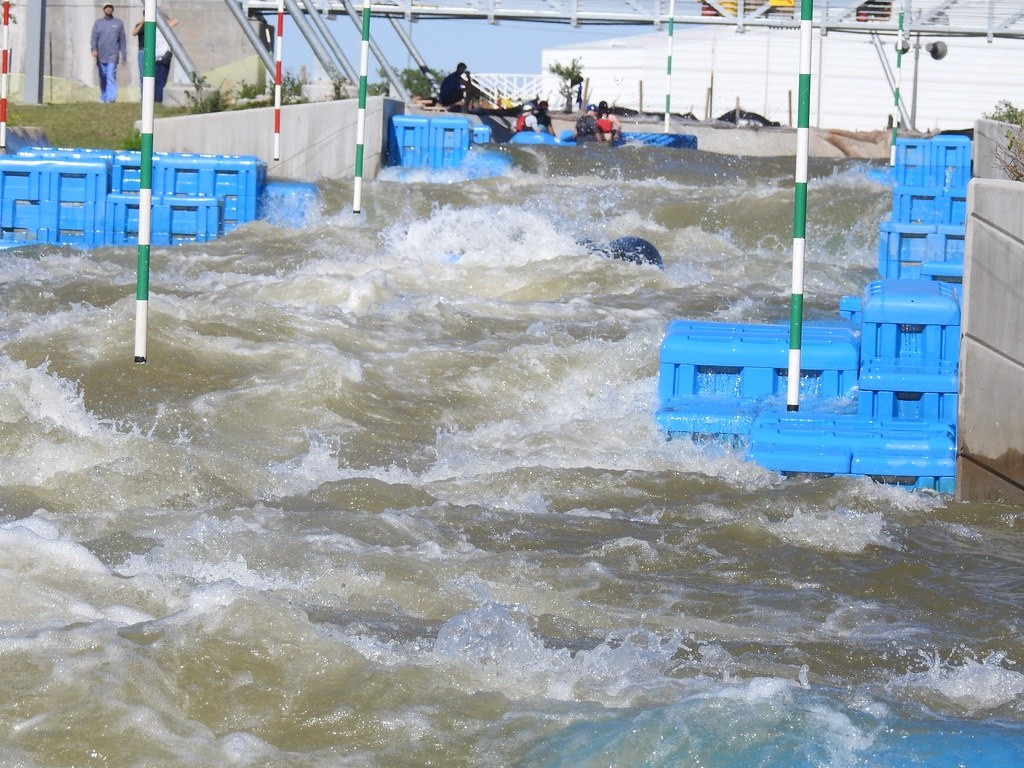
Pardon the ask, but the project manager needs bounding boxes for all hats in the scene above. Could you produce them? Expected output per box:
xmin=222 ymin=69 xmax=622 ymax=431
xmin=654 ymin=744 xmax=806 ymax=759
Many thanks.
xmin=523 ymin=103 xmax=537 ymax=110
xmin=587 ymin=105 xmax=600 ymax=112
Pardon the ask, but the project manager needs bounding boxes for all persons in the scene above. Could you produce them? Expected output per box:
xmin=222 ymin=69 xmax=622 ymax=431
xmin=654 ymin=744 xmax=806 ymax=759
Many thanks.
xmin=534 ymin=101 xmax=560 ymax=137
xmin=574 ymin=104 xmax=602 ymax=146
xmin=510 ymin=104 xmax=540 ymax=132
xmin=154 ymin=12 xmax=179 ymax=102
xmin=440 ymin=63 xmax=471 ymax=110
xmin=132 ymin=8 xmax=145 ymax=102
xmin=90 ymin=1 xmax=126 ymax=104
xmin=596 ymin=100 xmax=621 ymax=145
